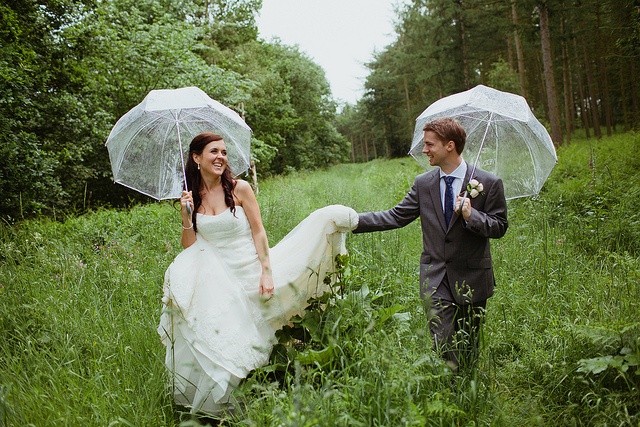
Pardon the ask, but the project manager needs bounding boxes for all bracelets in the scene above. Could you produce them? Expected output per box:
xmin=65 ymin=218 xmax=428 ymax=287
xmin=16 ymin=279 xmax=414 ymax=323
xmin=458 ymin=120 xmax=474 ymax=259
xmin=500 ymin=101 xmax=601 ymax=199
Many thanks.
xmin=182 ymin=223 xmax=194 ymax=230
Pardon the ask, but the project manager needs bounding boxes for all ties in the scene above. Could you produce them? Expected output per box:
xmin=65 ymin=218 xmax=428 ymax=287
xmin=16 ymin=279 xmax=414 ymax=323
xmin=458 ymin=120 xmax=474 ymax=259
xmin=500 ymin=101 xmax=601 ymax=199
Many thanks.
xmin=441 ymin=175 xmax=457 ymax=231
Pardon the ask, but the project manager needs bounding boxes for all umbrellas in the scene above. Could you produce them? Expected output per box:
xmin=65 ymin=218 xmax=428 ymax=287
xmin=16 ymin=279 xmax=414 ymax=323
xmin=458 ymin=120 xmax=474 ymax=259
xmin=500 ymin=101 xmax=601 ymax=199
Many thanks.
xmin=105 ymin=86 xmax=253 ymax=214
xmin=408 ymin=85 xmax=558 ymax=216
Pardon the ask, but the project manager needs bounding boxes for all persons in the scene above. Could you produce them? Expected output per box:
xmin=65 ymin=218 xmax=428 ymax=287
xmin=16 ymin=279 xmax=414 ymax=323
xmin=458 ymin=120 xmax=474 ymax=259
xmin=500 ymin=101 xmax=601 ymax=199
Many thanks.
xmin=155 ymin=132 xmax=275 ymax=423
xmin=352 ymin=117 xmax=509 ymax=374
